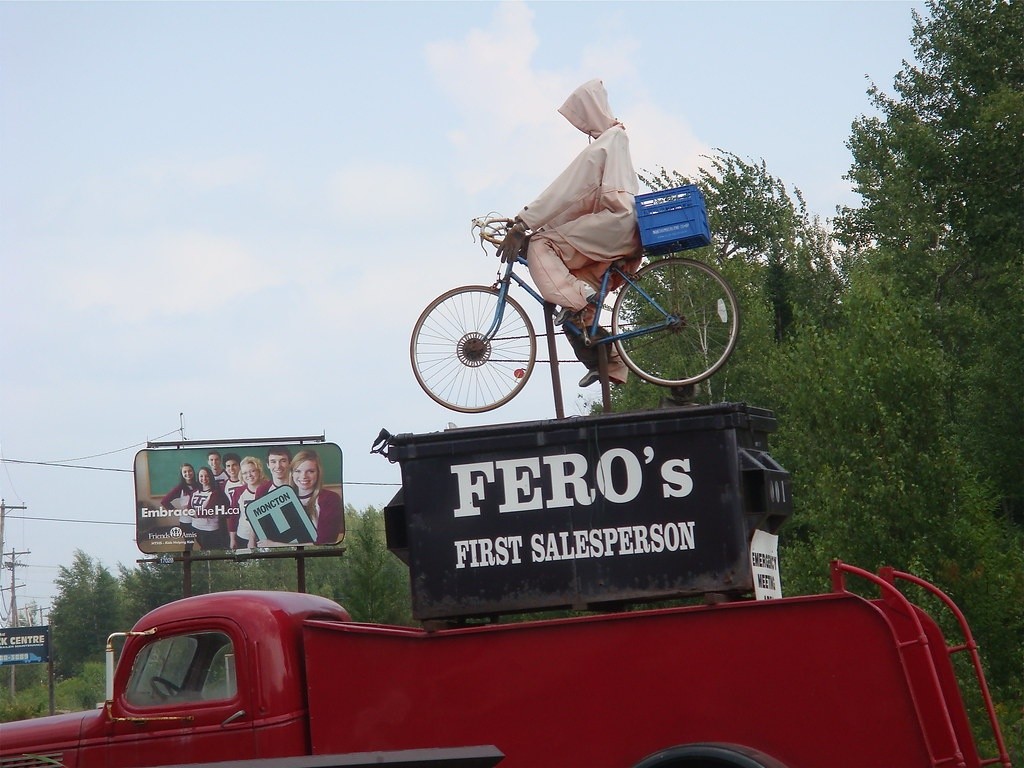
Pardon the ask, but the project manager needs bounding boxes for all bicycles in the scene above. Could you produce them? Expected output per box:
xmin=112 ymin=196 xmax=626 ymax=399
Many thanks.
xmin=411 ymin=213 xmax=740 ymax=414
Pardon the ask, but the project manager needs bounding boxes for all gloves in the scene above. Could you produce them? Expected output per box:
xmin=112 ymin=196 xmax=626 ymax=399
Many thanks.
xmin=496 ymin=223 xmax=540 ymax=264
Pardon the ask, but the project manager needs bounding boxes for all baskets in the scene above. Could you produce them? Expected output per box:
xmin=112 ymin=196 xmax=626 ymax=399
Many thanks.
xmin=634 ymin=184 xmax=711 ymax=255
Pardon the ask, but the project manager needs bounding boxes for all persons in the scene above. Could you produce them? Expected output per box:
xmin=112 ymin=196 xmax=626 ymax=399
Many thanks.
xmin=496 ymin=77 xmax=644 ymax=388
xmin=160 ymin=445 xmax=346 ymax=552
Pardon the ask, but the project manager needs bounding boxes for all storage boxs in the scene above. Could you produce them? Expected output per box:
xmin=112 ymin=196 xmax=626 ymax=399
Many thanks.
xmin=634 ymin=185 xmax=712 ymax=255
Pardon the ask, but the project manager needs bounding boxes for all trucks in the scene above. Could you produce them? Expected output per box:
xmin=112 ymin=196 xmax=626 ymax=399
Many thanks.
xmin=0 ymin=557 xmax=1012 ymax=768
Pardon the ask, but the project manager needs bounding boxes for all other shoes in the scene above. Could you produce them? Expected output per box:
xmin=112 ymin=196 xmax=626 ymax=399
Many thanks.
xmin=554 ymin=281 xmax=596 ymax=326
xmin=579 ymin=369 xmax=599 ymax=387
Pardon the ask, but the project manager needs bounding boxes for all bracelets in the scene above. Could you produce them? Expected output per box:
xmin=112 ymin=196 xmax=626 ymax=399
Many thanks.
xmin=519 ymin=221 xmax=526 ymax=232
